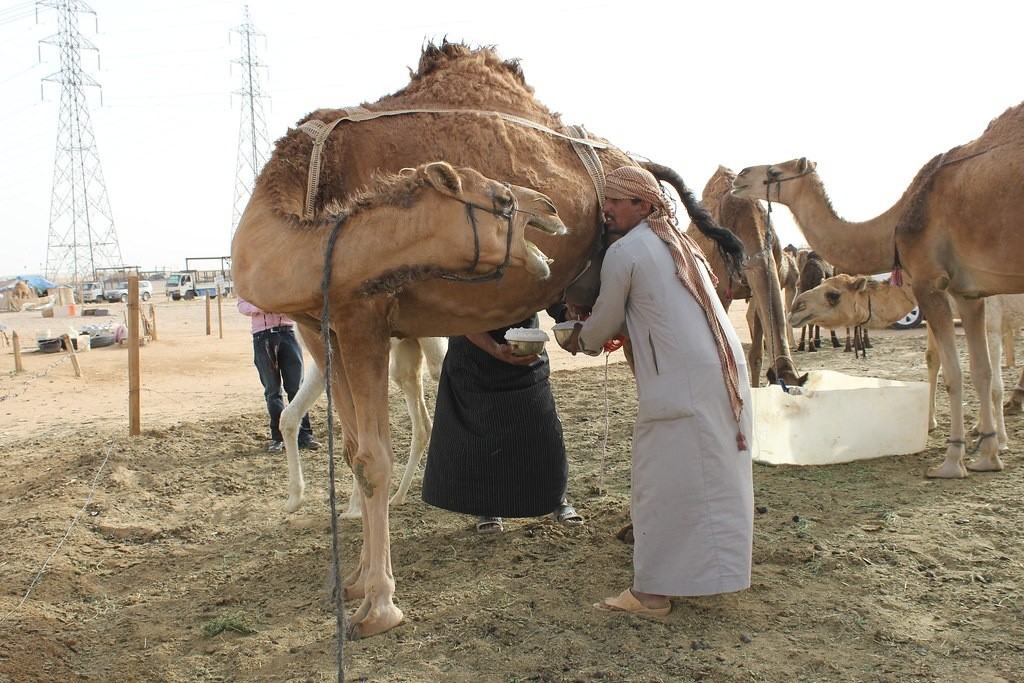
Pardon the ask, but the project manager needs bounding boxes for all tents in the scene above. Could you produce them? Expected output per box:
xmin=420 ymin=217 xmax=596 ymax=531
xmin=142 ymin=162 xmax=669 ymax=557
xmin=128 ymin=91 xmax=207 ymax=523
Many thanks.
xmin=0 ymin=274 xmax=67 ymax=313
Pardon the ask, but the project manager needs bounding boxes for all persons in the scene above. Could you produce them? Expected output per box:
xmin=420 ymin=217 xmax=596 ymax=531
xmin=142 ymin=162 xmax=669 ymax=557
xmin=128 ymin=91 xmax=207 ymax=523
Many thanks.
xmin=235 ymin=295 xmax=322 ymax=454
xmin=559 ymin=163 xmax=757 ymax=619
xmin=418 ymin=294 xmax=585 ymax=535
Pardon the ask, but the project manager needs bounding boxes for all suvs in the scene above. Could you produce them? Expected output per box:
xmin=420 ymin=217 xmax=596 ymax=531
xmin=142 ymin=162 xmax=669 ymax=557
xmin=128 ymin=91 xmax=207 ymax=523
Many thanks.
xmin=104 ymin=280 xmax=154 ymax=303
xmin=869 ymin=269 xmax=962 ymax=331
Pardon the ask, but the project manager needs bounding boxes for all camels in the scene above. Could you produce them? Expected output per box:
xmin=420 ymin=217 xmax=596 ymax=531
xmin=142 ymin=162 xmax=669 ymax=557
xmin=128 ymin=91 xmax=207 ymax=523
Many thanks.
xmin=685 ymin=100 xmax=1023 ymax=479
xmin=230 ymin=41 xmax=753 ymax=643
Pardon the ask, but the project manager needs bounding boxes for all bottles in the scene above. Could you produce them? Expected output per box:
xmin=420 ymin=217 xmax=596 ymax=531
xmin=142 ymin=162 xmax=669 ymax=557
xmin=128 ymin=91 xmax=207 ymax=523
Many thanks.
xmin=67 ymin=326 xmax=78 ymax=337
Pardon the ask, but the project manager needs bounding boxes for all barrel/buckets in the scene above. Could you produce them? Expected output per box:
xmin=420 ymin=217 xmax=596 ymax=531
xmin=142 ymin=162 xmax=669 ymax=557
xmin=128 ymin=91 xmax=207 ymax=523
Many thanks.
xmin=35 ymin=330 xmax=51 ymax=347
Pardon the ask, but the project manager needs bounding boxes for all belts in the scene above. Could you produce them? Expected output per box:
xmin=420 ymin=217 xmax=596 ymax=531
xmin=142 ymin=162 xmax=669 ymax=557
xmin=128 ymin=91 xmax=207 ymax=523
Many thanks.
xmin=253 ymin=326 xmax=295 ymax=339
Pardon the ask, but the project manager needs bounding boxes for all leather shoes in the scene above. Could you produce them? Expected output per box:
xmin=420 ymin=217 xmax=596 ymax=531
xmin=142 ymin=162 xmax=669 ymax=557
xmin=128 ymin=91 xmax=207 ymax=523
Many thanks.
xmin=268 ymin=440 xmax=285 ymax=453
xmin=300 ymin=434 xmax=322 ymax=449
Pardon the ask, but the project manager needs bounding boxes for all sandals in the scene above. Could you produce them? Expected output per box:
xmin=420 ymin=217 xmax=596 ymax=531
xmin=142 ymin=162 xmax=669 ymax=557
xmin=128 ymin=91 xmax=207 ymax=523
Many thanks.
xmin=544 ymin=502 xmax=583 ymax=526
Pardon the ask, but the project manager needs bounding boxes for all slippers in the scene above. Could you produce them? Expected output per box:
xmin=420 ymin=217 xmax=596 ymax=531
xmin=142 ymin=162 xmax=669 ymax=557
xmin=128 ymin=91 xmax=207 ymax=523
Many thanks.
xmin=476 ymin=514 xmax=503 ymax=533
xmin=592 ymin=587 xmax=672 ymax=617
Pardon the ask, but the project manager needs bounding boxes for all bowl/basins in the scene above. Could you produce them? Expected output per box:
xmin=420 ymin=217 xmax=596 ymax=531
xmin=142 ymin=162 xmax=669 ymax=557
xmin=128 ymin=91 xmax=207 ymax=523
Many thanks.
xmin=552 ymin=327 xmax=581 ymax=352
xmin=507 ymin=339 xmax=544 ymax=356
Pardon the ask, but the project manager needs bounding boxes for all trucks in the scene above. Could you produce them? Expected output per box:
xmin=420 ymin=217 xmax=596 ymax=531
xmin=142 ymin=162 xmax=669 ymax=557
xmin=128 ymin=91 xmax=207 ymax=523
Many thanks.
xmin=75 ymin=280 xmax=105 ymax=304
xmin=165 ymin=271 xmax=234 ymax=302
